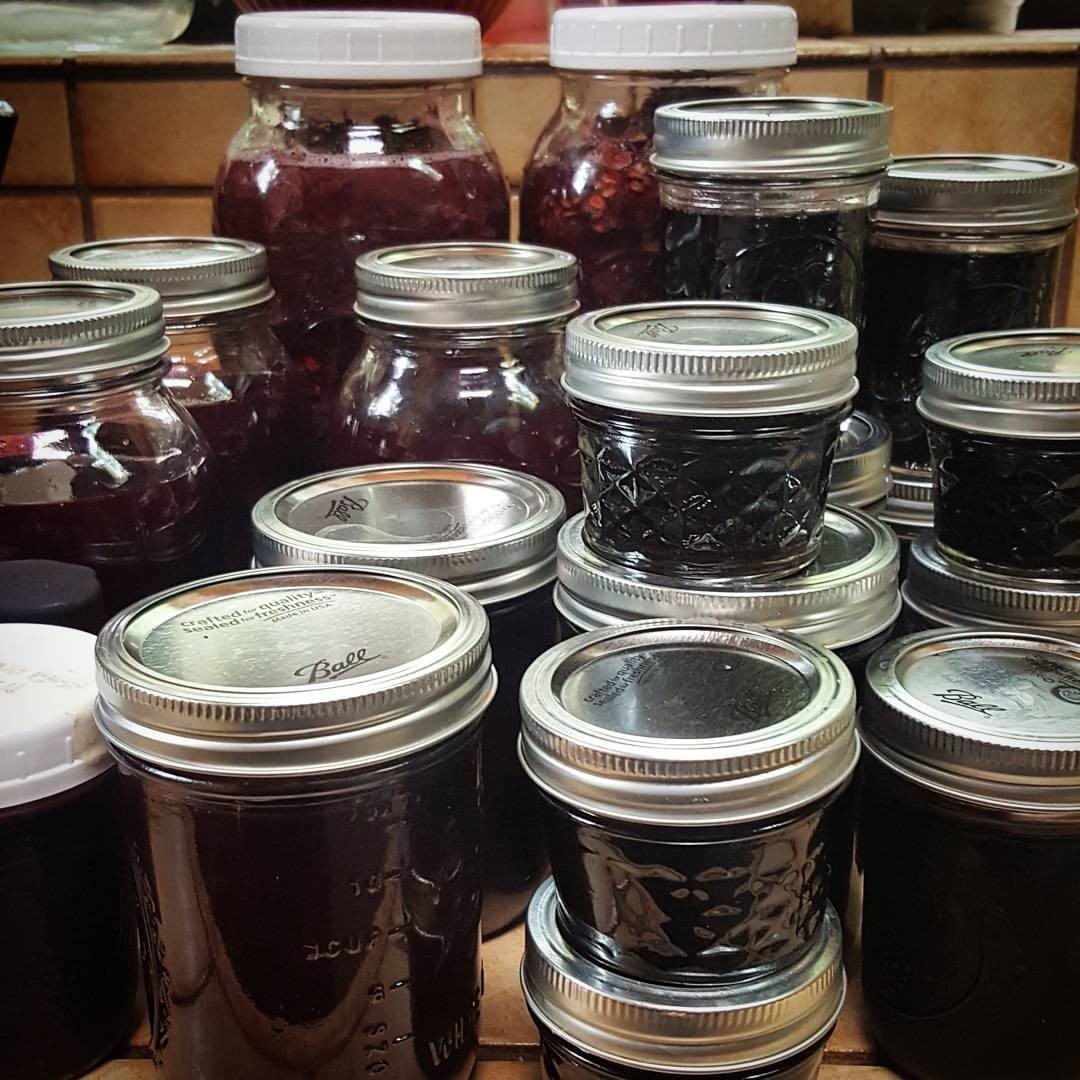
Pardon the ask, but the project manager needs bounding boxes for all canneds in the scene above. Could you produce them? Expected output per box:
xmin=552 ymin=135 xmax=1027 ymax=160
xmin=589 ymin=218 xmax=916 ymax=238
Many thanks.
xmin=0 ymin=6 xmax=1080 ymax=1080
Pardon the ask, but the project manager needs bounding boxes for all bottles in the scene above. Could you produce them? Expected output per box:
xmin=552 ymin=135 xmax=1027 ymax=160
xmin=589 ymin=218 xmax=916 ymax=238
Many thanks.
xmin=0 ymin=6 xmax=1078 ymax=1080
